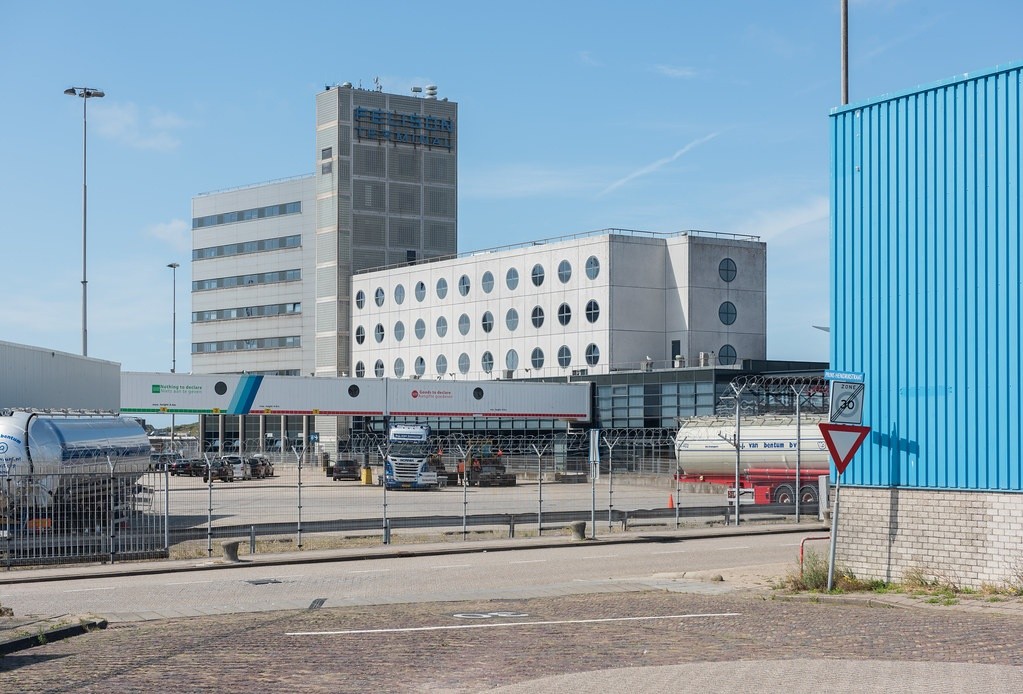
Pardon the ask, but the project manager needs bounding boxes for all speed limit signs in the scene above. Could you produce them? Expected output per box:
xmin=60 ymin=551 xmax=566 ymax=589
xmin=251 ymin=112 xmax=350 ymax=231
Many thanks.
xmin=830 ymin=380 xmax=865 ymax=424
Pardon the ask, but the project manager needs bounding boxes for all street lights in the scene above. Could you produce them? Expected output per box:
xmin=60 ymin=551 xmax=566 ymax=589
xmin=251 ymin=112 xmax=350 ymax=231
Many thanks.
xmin=63 ymin=87 xmax=105 ymax=357
xmin=167 ymin=263 xmax=181 ymax=371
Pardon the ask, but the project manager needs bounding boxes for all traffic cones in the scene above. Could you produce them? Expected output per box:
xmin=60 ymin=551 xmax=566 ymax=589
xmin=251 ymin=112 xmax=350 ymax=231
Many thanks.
xmin=668 ymin=494 xmax=675 ymax=510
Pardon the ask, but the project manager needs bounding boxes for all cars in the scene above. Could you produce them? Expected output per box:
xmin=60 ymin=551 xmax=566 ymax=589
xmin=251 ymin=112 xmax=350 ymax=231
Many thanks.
xmin=326 ymin=459 xmax=362 ymax=481
xmin=150 ymin=452 xmax=274 ymax=483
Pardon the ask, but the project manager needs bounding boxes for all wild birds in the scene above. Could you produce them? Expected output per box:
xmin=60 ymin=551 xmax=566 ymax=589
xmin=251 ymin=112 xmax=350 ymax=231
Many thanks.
xmin=342 ymin=372 xmax=348 ymax=377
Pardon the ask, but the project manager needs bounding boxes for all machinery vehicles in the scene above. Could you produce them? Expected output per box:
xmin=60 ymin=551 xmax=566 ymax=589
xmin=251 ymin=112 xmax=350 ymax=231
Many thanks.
xmin=459 ymin=439 xmax=517 ymax=487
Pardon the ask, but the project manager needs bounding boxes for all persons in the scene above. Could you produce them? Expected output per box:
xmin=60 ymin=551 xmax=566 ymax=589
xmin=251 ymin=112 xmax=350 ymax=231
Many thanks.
xmin=436 ymin=449 xmax=443 ymax=457
xmin=472 ymin=458 xmax=481 ymax=472
xmin=457 ymin=459 xmax=467 ymax=486
xmin=497 ymin=448 xmax=503 ymax=460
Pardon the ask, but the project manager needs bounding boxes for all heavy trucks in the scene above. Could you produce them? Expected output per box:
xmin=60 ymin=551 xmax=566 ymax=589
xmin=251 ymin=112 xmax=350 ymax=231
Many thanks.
xmin=672 ymin=412 xmax=829 ymax=515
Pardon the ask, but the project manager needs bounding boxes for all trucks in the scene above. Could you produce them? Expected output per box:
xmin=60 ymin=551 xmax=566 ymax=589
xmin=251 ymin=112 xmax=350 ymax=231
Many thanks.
xmin=385 ymin=425 xmax=435 ymax=490
